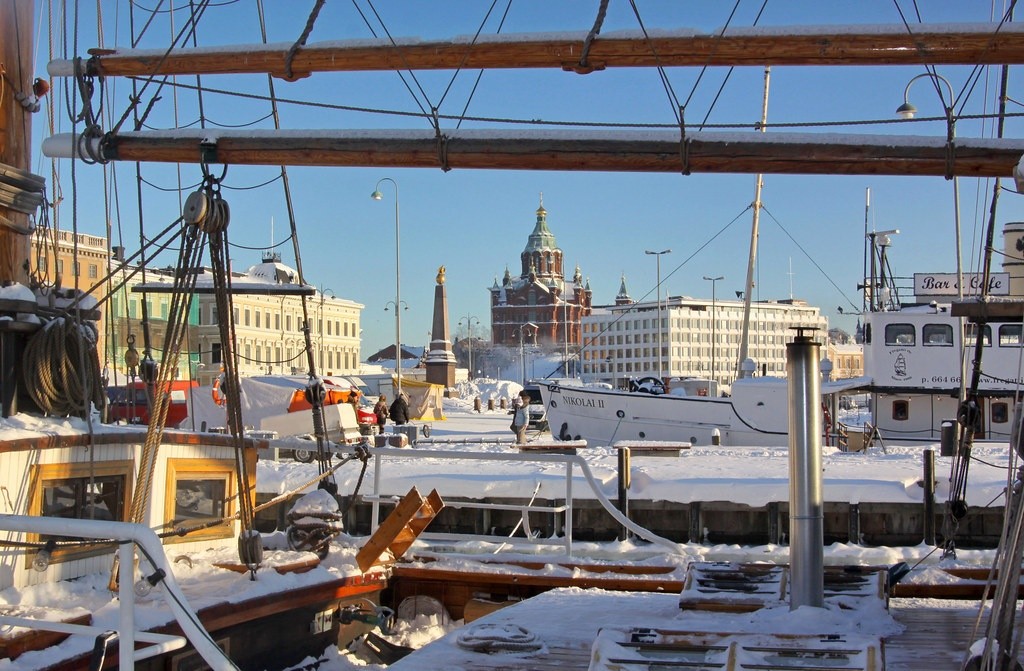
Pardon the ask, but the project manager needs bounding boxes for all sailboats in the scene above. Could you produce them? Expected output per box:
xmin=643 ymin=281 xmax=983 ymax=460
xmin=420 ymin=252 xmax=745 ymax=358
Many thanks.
xmin=0 ymin=1 xmax=450 ymax=671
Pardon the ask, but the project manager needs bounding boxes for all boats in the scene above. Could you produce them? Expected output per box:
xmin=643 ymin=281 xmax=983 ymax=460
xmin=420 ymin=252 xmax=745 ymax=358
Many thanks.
xmin=542 ymin=149 xmax=1023 ymax=452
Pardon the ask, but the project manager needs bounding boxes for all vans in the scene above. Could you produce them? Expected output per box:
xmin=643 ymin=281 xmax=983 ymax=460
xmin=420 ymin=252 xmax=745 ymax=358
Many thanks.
xmin=321 ymin=375 xmax=379 ymax=426
xmin=98 ymin=378 xmax=202 ymax=428
xmin=340 ymin=376 xmax=380 ymax=407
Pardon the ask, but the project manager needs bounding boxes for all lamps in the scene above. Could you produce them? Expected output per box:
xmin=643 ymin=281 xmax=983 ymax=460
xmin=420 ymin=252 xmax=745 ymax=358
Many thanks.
xmin=928 ymin=300 xmax=938 ymax=315
xmin=711 ymin=428 xmax=721 ymax=446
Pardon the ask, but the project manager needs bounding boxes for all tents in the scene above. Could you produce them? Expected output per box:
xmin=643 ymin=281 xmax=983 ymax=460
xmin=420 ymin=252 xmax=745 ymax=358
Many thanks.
xmin=393 ymin=379 xmax=446 ymax=422
xmin=179 ymin=375 xmax=359 ymax=431
xmin=360 ymin=344 xmax=426 ymax=369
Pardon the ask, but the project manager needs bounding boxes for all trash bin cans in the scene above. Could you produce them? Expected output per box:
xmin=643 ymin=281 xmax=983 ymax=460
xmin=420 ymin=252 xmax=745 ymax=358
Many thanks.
xmin=941 ymin=419 xmax=958 ymax=456
xmin=359 ymin=424 xmax=380 ymax=442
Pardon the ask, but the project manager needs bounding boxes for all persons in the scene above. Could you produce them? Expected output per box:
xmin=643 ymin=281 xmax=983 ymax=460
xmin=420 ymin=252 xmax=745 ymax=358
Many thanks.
xmin=373 ymin=394 xmax=390 ymax=435
xmin=508 ymin=389 xmax=530 ymax=444
xmin=389 ymin=391 xmax=410 ymax=426
xmin=349 ymin=389 xmax=371 ymax=423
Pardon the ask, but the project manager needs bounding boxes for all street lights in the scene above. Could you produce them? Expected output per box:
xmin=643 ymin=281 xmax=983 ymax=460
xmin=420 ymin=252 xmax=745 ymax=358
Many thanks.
xmin=895 ymin=74 xmax=970 ymax=454
xmin=369 ymin=177 xmax=402 ymax=402
xmin=308 ymin=288 xmax=336 ymax=371
xmin=510 ymin=327 xmax=532 ymax=387
xmin=644 ymin=249 xmax=673 ymax=380
xmin=384 ymin=300 xmax=409 ymax=381
xmin=703 ymin=276 xmax=723 ymax=383
xmin=457 ymin=315 xmax=480 ymax=380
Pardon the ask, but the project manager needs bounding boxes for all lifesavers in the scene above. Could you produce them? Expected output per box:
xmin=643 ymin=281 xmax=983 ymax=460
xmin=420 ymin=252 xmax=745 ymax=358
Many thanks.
xmin=211 ymin=371 xmax=243 ymax=407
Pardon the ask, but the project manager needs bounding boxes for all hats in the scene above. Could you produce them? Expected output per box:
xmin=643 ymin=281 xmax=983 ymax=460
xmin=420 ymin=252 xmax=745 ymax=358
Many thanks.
xmin=350 ymin=391 xmax=357 ymax=397
xmin=515 ymin=396 xmax=523 ymax=406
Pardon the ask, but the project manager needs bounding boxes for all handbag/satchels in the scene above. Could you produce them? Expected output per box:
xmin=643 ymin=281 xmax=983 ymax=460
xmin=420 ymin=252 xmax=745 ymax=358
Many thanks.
xmin=378 ymin=412 xmax=386 ymax=418
